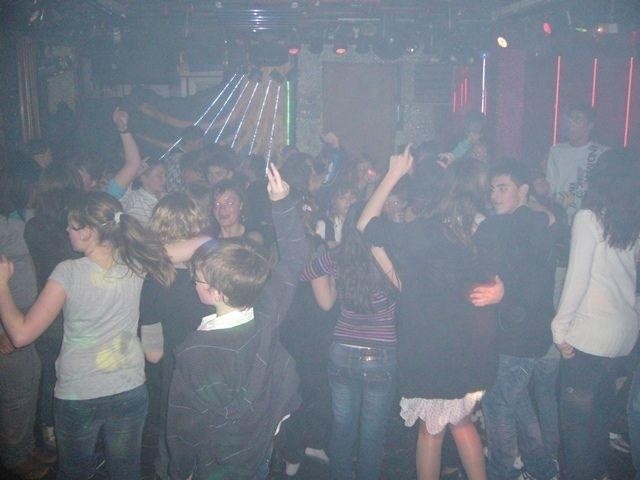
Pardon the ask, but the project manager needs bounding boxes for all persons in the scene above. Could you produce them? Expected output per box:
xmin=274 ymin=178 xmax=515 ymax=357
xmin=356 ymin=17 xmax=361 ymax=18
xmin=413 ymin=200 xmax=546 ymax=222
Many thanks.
xmin=1 ymin=103 xmax=639 ymax=480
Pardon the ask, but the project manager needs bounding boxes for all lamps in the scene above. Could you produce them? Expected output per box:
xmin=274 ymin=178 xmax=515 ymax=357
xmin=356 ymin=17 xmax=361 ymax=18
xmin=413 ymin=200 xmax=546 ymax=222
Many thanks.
xmin=280 ymin=3 xmax=626 ymax=59
